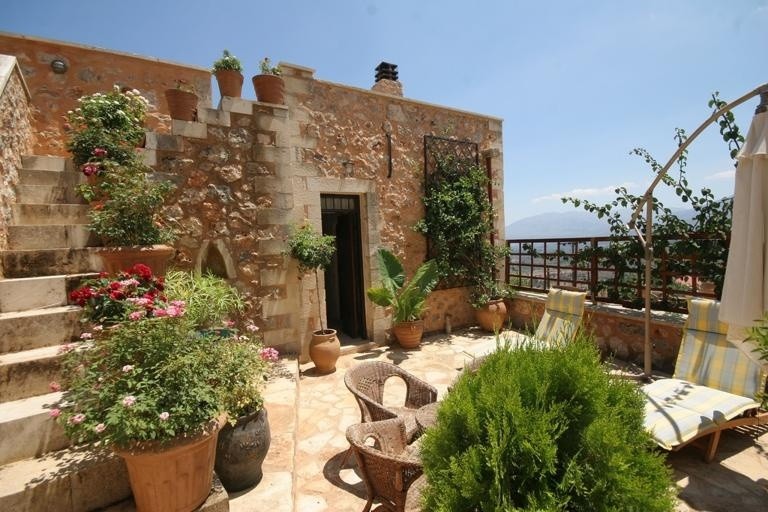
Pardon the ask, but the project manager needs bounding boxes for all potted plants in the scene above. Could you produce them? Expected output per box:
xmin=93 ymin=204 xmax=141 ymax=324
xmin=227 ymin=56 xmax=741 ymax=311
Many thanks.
xmin=212 ymin=50 xmax=286 ymax=103
xmin=299 ymin=227 xmax=519 ymax=375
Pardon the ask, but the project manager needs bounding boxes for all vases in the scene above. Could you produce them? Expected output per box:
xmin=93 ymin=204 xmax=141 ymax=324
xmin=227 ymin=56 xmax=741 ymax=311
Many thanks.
xmin=93 ymin=247 xmax=175 ymax=284
xmin=111 ymin=406 xmax=271 ymax=512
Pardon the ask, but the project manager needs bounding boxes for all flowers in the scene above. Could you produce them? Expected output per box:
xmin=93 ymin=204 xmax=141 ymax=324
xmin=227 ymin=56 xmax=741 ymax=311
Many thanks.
xmin=59 ymin=81 xmax=175 ymax=247
xmin=50 ymin=262 xmax=277 ymax=435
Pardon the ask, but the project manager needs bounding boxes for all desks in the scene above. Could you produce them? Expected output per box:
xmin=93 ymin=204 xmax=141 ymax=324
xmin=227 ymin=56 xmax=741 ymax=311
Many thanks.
xmin=414 ymin=401 xmax=440 ymax=436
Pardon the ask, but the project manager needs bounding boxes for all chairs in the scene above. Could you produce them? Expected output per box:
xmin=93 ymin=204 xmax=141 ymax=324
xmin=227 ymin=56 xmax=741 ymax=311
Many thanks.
xmin=405 ymin=472 xmax=431 ymax=511
xmin=342 ymin=360 xmax=439 ymax=444
xmin=345 ymin=417 xmax=424 ymax=511
xmin=625 ymin=293 xmax=763 ymax=464
xmin=529 ymin=290 xmax=587 ymax=352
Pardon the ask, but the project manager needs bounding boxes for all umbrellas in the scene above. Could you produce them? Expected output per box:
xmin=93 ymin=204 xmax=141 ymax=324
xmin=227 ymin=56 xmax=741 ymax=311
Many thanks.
xmin=627 ymin=81 xmax=767 ymax=378
